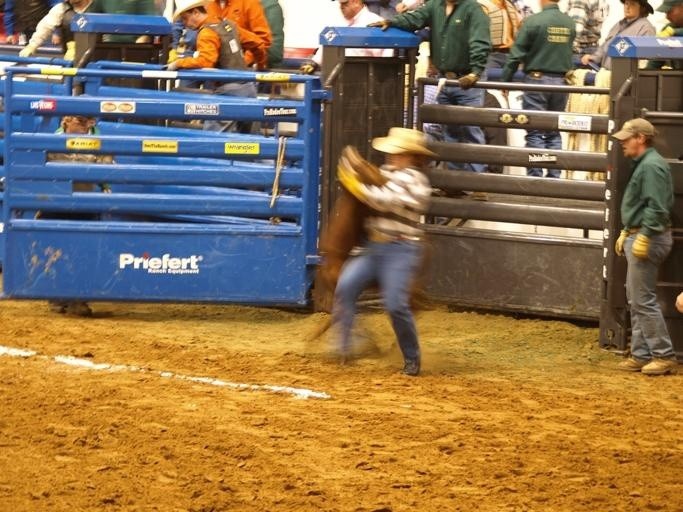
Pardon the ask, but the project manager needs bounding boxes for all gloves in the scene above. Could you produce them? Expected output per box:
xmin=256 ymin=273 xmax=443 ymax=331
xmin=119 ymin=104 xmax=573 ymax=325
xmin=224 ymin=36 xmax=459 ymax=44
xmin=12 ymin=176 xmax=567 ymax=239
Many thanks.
xmin=631 ymin=234 xmax=652 ymax=262
xmin=458 ymin=74 xmax=479 ymax=91
xmin=614 ymin=230 xmax=628 ymax=257
xmin=62 ymin=42 xmax=78 ymax=61
xmin=17 ymin=44 xmax=37 ymax=58
xmin=365 ymin=19 xmax=392 ymax=31
xmin=299 ymin=59 xmax=320 ymax=77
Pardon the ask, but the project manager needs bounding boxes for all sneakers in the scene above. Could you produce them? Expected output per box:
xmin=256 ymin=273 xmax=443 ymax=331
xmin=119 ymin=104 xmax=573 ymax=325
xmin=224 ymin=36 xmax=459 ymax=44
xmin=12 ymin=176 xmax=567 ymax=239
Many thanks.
xmin=50 ymin=299 xmax=94 ymax=316
xmin=640 ymin=358 xmax=678 ymax=375
xmin=618 ymin=357 xmax=648 ymax=372
xmin=401 ymin=357 xmax=421 ymax=377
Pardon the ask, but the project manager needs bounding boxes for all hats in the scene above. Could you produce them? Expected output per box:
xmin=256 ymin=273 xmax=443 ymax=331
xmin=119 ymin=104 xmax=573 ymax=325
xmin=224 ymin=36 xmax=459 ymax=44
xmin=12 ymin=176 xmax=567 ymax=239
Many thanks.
xmin=371 ymin=125 xmax=442 ymax=160
xmin=656 ymin=0 xmax=683 ymax=14
xmin=172 ymin=0 xmax=207 ymax=26
xmin=611 ymin=117 xmax=656 ymax=141
xmin=619 ymin=0 xmax=655 ymax=15
